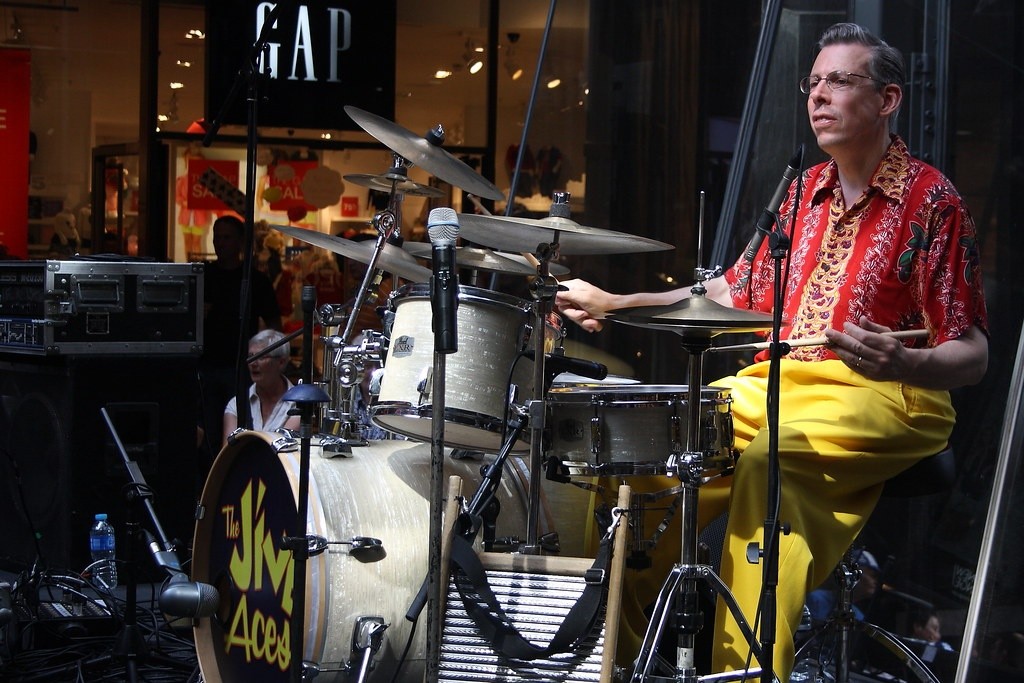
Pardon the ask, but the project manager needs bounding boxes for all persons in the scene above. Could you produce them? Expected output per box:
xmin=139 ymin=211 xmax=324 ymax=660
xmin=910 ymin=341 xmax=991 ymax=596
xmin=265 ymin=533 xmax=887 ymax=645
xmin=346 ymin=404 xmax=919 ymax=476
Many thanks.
xmin=904 ymin=608 xmax=944 ymax=644
xmin=553 ymin=24 xmax=993 ymax=683
xmin=222 ymin=329 xmax=302 ymax=441
xmin=805 ymin=549 xmax=882 ymax=653
xmin=190 ymin=215 xmax=281 ymax=446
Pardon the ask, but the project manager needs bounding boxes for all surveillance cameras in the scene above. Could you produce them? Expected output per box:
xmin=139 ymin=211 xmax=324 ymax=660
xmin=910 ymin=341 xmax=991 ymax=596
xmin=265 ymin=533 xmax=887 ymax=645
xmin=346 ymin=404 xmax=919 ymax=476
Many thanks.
xmin=508 ymin=34 xmax=520 ymax=42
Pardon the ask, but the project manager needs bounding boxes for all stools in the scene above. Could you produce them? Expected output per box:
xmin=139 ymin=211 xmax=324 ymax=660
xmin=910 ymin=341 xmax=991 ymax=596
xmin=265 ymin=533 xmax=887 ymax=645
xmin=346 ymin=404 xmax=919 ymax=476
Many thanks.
xmin=782 ymin=445 xmax=953 ymax=683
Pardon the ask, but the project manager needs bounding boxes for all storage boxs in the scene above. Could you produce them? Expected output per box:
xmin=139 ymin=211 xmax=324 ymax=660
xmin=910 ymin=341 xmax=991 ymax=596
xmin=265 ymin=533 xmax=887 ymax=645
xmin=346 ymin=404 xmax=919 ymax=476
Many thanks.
xmin=1 ymin=258 xmax=207 ymax=356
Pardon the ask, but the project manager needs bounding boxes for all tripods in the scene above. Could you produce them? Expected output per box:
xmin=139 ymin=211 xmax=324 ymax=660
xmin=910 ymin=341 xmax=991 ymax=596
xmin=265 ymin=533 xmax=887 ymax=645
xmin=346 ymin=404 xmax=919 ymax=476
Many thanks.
xmin=17 ymin=406 xmax=198 ymax=683
xmin=630 ymin=338 xmax=783 ymax=683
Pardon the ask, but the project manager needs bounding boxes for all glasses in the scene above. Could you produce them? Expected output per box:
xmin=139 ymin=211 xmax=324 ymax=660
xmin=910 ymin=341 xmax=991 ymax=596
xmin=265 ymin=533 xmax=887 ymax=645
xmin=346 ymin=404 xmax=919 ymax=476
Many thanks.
xmin=800 ymin=70 xmax=886 ymax=94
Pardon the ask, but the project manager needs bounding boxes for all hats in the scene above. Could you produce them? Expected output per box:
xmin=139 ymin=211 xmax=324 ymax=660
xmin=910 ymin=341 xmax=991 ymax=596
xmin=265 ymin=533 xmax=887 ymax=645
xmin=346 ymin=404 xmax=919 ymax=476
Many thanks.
xmin=832 ymin=548 xmax=882 ymax=585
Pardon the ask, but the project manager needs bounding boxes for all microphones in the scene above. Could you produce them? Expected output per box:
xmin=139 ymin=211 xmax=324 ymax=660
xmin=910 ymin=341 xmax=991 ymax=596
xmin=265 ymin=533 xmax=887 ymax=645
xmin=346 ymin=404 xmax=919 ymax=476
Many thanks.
xmin=744 ymin=150 xmax=803 ymax=263
xmin=427 ymin=208 xmax=460 ymax=354
xmin=525 ymin=351 xmax=608 ymax=381
xmin=158 ymin=573 xmax=220 ymax=619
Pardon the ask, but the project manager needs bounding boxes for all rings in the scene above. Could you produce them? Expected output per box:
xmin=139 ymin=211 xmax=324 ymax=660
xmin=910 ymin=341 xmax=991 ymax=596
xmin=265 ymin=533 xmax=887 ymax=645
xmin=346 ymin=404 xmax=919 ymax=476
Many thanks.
xmin=857 ymin=356 xmax=862 ymax=367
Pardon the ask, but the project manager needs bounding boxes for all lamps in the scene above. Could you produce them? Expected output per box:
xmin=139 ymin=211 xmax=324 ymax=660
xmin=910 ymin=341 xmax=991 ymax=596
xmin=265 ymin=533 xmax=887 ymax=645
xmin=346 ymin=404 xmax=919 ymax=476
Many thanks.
xmin=462 ymin=29 xmax=483 ymax=74
xmin=502 ymin=41 xmax=522 ymax=80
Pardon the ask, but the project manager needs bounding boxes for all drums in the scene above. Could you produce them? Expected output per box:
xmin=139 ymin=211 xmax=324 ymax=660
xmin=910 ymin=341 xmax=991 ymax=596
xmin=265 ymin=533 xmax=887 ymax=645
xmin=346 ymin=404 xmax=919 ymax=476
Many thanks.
xmin=374 ymin=279 xmax=564 ymax=458
xmin=187 ymin=424 xmax=556 ymax=682
xmin=546 ymin=382 xmax=734 ymax=479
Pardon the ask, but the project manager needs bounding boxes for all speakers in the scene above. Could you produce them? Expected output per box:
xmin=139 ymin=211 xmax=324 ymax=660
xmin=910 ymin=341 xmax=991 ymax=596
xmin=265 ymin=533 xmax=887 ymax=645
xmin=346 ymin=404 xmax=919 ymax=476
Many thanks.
xmin=0 ymin=353 xmax=201 ymax=580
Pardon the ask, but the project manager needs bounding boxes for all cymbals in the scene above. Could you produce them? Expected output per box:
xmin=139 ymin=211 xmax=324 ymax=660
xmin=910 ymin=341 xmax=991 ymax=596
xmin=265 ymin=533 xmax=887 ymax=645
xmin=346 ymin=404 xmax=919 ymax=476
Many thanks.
xmin=364 ymin=237 xmax=573 ymax=278
xmin=604 ymin=294 xmax=784 ymax=336
xmin=265 ymin=224 xmax=434 ymax=285
xmin=456 ymin=211 xmax=677 ymax=256
xmin=341 ymin=103 xmax=508 ymax=202
xmin=343 ymin=173 xmax=445 ymax=199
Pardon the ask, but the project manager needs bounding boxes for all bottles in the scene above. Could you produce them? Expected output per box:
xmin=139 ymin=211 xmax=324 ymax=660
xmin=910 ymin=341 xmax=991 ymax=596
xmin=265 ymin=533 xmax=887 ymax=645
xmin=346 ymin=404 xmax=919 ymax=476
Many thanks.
xmin=790 ymin=604 xmax=812 ymax=679
xmin=90 ymin=513 xmax=117 ymax=589
